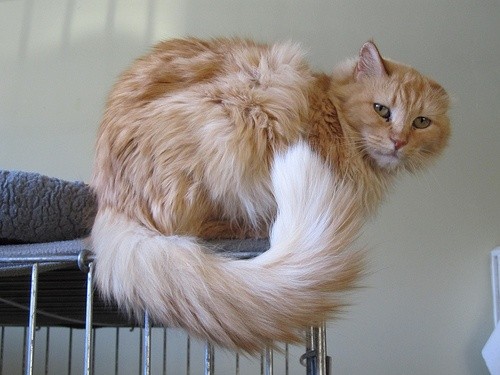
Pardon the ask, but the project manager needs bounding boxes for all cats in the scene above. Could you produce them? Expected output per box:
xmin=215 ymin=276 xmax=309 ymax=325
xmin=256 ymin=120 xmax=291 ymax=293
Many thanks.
xmin=89 ymin=31 xmax=449 ymax=355
